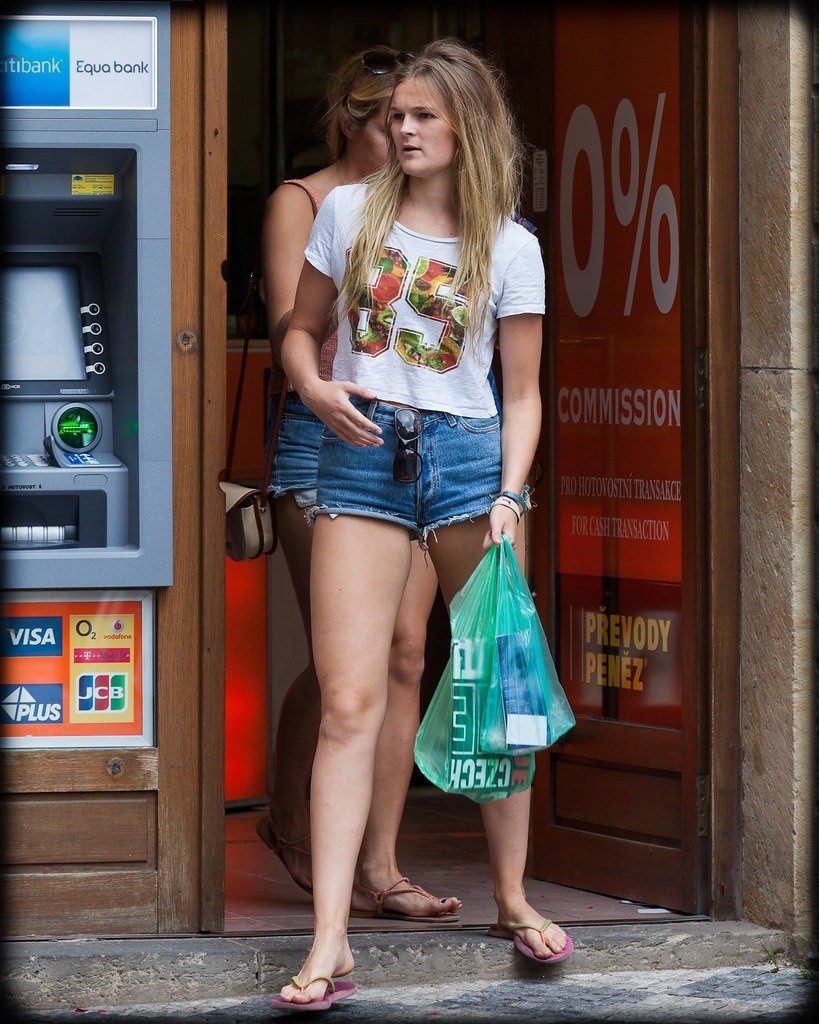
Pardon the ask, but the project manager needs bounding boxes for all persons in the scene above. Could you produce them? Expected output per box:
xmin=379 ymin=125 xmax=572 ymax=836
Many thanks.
xmin=256 ymin=47 xmax=464 ymax=924
xmin=269 ymin=43 xmax=571 ymax=1009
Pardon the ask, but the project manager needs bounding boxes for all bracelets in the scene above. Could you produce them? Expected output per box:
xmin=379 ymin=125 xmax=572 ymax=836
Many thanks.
xmin=490 ymin=490 xmax=526 ymax=525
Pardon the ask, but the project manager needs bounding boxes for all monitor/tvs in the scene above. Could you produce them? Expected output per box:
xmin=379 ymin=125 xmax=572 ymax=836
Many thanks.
xmin=0 ymin=263 xmax=90 ymax=380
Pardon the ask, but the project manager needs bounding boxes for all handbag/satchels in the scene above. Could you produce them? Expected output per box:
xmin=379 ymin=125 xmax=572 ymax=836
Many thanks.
xmin=219 ymin=481 xmax=277 ymax=562
xmin=413 ymin=533 xmax=575 ymax=807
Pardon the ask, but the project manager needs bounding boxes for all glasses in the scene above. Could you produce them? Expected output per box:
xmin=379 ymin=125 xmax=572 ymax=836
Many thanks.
xmin=343 ymin=49 xmax=417 ymax=106
xmin=393 ymin=406 xmax=425 ymax=483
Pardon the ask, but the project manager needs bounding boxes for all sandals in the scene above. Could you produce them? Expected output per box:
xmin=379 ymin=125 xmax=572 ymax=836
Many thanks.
xmin=257 ymin=815 xmax=315 ymax=894
xmin=350 ymin=877 xmax=461 ymax=923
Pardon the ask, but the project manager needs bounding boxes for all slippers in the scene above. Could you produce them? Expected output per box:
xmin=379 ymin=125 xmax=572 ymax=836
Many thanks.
xmin=488 ymin=919 xmax=573 ymax=965
xmin=270 ymin=976 xmax=357 ymax=1010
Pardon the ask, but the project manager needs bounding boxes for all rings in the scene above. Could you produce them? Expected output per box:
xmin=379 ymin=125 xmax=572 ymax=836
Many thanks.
xmin=349 ymin=433 xmax=354 ymax=439
